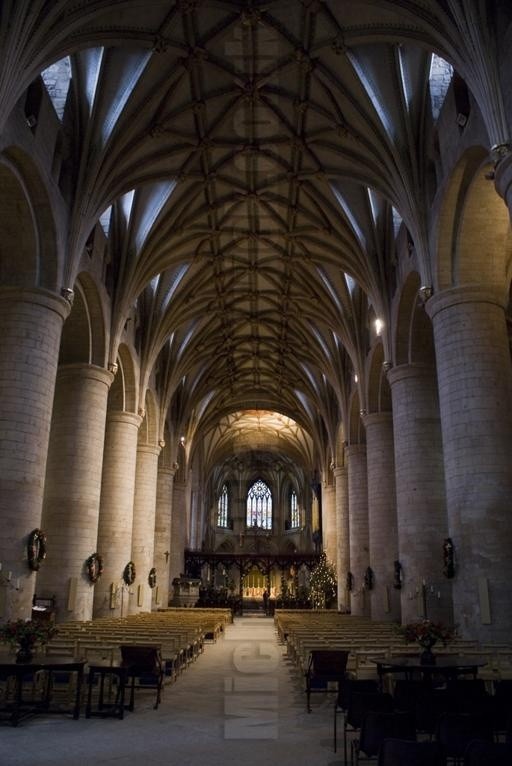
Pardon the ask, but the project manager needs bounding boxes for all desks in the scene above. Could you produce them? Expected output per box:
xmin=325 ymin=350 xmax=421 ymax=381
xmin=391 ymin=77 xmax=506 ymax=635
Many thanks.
xmin=0 ymin=653 xmax=87 ymax=727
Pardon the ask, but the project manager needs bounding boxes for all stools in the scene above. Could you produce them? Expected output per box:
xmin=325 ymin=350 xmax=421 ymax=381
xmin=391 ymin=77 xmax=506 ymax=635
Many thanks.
xmin=85 ymin=661 xmax=129 ymax=720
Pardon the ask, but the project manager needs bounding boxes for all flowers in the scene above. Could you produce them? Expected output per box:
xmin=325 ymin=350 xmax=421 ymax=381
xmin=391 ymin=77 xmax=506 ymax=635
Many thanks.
xmin=0 ymin=618 xmax=59 ymax=650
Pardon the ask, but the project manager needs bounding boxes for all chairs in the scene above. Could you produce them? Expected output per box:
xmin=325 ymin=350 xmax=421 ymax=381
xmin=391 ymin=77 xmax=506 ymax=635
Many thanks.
xmin=294 ymin=650 xmax=511 ymax=766
xmin=117 ymin=645 xmax=167 ymax=712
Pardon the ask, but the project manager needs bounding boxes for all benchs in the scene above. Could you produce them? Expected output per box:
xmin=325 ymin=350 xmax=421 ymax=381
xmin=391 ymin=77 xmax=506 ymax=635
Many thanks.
xmin=37 ymin=605 xmax=230 ymax=687
xmin=272 ymin=608 xmax=511 ymax=695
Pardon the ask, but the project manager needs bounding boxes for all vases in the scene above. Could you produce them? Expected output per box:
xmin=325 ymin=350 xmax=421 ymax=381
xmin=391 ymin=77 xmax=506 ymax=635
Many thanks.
xmin=14 ymin=648 xmax=33 ymax=664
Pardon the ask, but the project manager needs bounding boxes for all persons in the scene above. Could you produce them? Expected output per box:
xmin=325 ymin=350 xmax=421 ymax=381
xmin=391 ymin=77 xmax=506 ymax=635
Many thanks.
xmin=263 ymin=591 xmax=268 ymax=609
